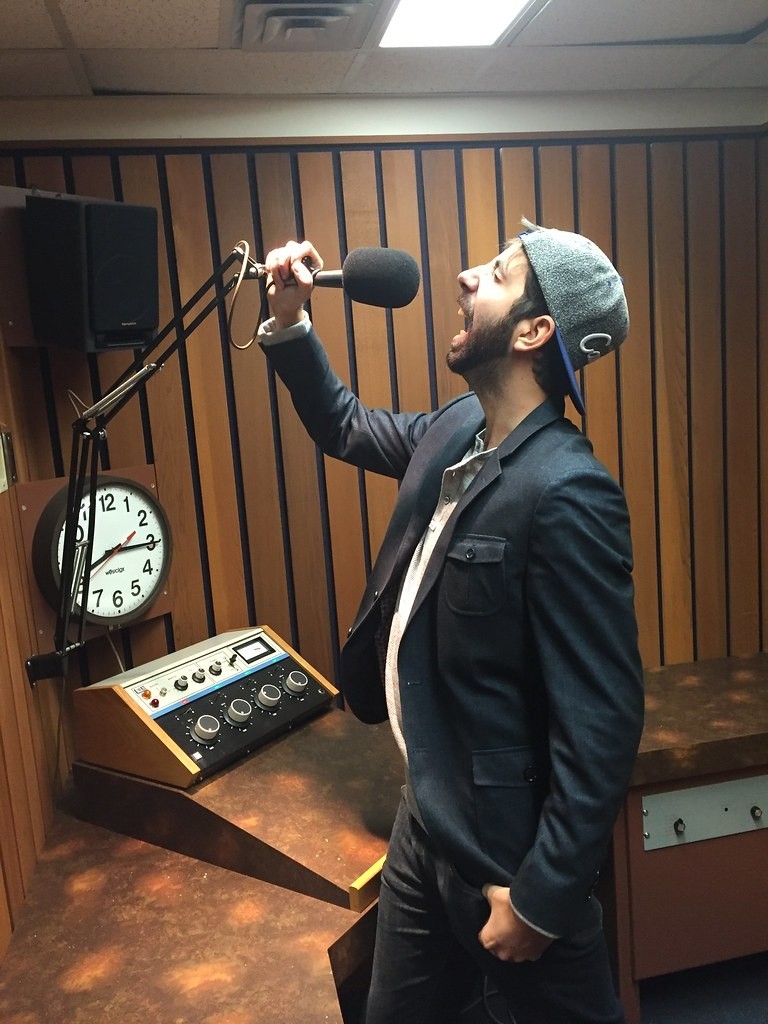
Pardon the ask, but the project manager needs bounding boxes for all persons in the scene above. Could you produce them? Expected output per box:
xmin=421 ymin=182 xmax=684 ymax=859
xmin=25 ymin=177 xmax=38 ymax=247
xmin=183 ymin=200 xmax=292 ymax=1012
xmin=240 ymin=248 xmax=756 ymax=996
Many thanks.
xmin=256 ymin=217 xmax=645 ymax=1024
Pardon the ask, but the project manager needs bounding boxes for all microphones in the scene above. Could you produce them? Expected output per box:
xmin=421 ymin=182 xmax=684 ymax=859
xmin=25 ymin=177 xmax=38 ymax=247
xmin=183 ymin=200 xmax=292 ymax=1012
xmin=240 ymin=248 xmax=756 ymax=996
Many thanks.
xmin=280 ymin=246 xmax=422 ymax=309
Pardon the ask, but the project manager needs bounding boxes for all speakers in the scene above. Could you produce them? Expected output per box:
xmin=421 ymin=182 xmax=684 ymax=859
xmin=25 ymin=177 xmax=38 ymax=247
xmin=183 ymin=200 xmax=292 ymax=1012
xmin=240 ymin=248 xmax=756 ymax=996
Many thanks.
xmin=25 ymin=195 xmax=159 ymax=350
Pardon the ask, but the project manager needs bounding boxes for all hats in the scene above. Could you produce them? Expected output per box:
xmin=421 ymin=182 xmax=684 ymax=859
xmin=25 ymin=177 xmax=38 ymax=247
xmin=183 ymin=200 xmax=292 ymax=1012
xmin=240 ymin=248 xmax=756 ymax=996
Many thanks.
xmin=521 ymin=228 xmax=629 ymax=417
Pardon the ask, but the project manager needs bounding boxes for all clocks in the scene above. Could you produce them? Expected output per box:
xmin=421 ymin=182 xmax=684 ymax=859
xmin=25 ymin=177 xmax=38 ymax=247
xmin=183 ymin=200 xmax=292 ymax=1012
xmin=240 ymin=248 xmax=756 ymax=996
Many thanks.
xmin=32 ymin=473 xmax=174 ymax=626
